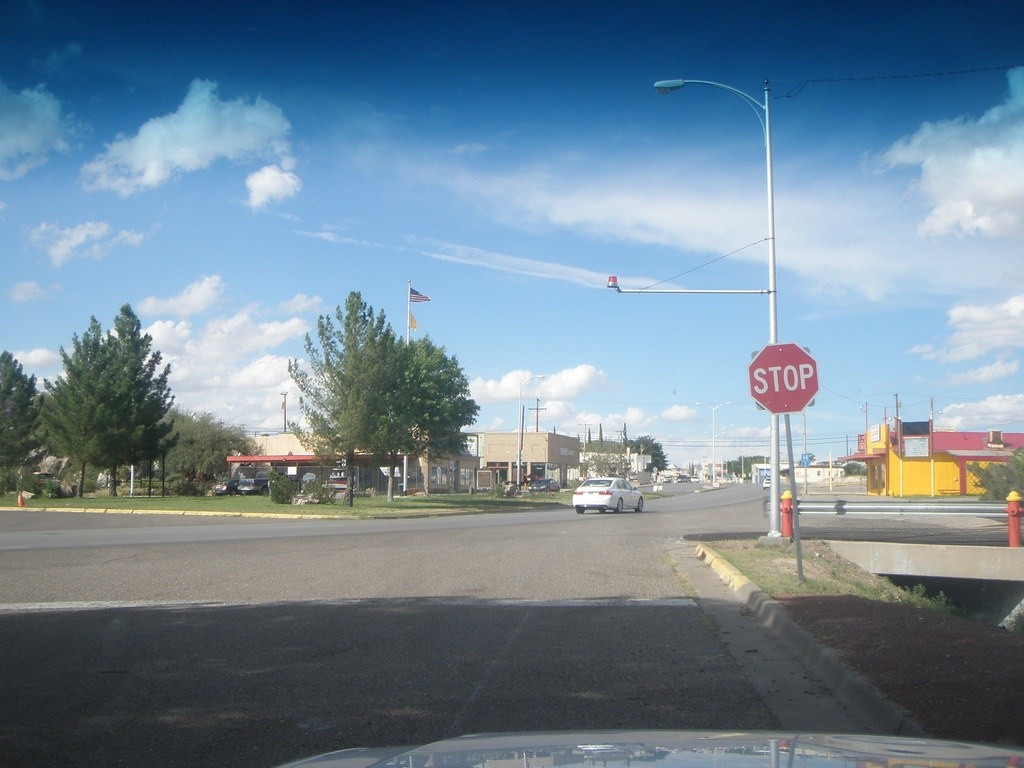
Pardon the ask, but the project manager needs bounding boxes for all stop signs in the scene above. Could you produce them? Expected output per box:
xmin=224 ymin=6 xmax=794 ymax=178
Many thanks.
xmin=749 ymin=343 xmax=820 ymax=415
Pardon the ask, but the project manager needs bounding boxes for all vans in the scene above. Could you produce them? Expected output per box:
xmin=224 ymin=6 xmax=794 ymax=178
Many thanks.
xmin=32 ymin=471 xmax=55 ymax=487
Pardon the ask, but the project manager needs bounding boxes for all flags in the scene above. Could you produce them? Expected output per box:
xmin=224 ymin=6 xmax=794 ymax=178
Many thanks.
xmin=410 ymin=287 xmax=431 ymax=302
xmin=411 ymin=315 xmax=416 ymax=331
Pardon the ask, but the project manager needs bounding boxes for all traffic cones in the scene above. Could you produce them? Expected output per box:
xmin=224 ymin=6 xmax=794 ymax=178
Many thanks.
xmin=17 ymin=491 xmax=28 ymax=507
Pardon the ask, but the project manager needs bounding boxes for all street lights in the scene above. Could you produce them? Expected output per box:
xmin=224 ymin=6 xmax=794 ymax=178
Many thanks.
xmin=708 ymin=423 xmax=735 ymax=483
xmin=651 ymin=74 xmax=779 ymax=544
xmin=695 ymin=400 xmax=733 ymax=489
xmin=516 ymin=375 xmax=545 ymax=492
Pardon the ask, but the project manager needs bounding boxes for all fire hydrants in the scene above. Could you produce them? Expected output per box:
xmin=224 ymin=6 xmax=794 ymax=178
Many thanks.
xmin=780 ymin=489 xmax=797 ymax=539
xmin=1005 ymin=490 xmax=1024 ymax=547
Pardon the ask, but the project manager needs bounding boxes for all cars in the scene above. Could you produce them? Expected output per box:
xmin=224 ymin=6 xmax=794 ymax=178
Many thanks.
xmin=572 ymin=477 xmax=644 ymax=515
xmin=97 ymin=471 xmax=123 ymax=490
xmin=650 ymin=471 xmax=700 ymax=484
xmin=528 ymin=479 xmax=561 ymax=494
xmin=762 ymin=478 xmax=771 ymax=490
xmin=211 ymin=471 xmax=303 ymax=497
